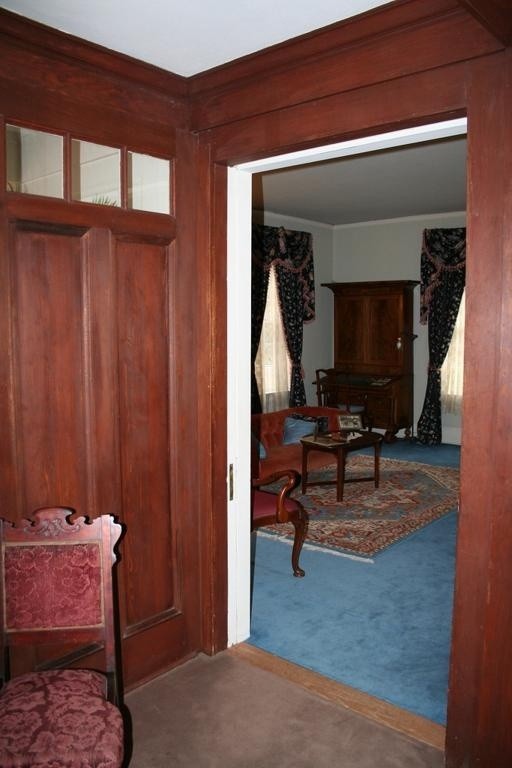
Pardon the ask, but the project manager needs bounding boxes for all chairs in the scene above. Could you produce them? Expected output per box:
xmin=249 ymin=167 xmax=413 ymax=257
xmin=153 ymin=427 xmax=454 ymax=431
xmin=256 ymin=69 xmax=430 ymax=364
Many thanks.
xmin=251 ymin=470 xmax=305 ymax=577
xmin=1 ymin=507 xmax=133 ymax=768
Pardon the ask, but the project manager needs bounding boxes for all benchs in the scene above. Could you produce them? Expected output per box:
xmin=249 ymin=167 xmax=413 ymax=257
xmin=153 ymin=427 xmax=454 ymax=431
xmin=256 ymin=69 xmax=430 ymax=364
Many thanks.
xmin=251 ymin=407 xmax=351 ymax=480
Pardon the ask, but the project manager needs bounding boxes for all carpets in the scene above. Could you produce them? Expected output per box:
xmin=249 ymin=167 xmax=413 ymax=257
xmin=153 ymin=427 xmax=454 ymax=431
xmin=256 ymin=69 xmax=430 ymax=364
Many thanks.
xmin=254 ymin=453 xmax=461 ymax=565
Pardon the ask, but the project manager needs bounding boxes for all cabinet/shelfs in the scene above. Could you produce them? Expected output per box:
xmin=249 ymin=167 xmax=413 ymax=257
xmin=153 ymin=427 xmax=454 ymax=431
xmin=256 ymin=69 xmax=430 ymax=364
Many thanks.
xmin=312 ymin=279 xmax=421 ymax=444
